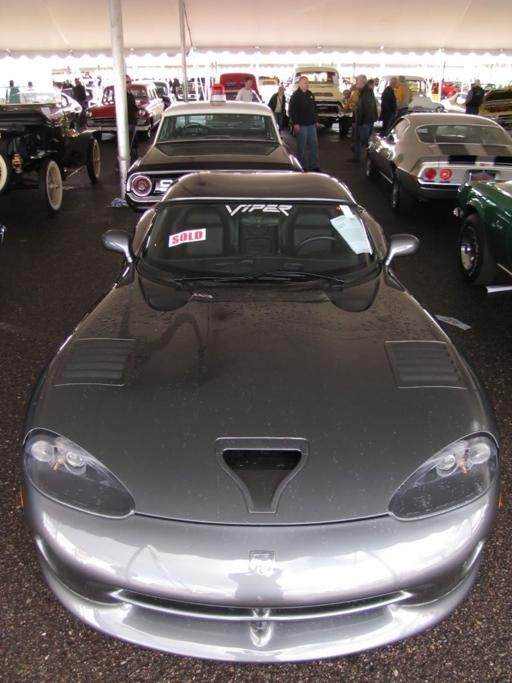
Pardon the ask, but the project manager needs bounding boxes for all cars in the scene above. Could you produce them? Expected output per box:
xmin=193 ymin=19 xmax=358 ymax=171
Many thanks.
xmin=86 ymin=83 xmax=165 ymax=139
xmin=479 ymin=89 xmax=511 ymax=126
xmin=3 ymin=91 xmax=83 ymax=135
xmin=125 ymin=83 xmax=304 ymax=213
xmin=141 ymin=79 xmax=205 ymax=107
xmin=376 ymin=75 xmax=446 ymax=112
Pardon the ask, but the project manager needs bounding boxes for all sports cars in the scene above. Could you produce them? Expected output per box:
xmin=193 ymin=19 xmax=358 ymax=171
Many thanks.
xmin=21 ymin=168 xmax=500 ymax=664
xmin=366 ymin=111 xmax=512 ymax=214
xmin=453 ymin=167 xmax=512 ymax=304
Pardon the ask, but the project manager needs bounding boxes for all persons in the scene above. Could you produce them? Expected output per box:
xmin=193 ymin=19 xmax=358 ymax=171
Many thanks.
xmin=5 ymin=80 xmax=20 ymax=103
xmin=269 ymin=86 xmax=286 ymax=130
xmin=344 ymin=74 xmax=413 ymax=163
xmin=236 ymin=77 xmax=253 ymax=103
xmin=113 ymin=76 xmax=140 ymax=171
xmin=24 ymin=81 xmax=36 ymax=103
xmin=169 ymin=77 xmax=181 ymax=94
xmin=288 ymin=77 xmax=322 ymax=172
xmin=62 ymin=77 xmax=86 ymax=108
xmin=465 ymin=79 xmax=486 ymax=115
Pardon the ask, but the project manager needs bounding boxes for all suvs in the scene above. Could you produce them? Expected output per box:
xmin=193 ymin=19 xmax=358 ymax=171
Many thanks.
xmin=219 ymin=73 xmax=261 ymax=99
xmin=287 ymin=66 xmax=351 ymax=129
xmin=0 ymin=88 xmax=103 ymax=215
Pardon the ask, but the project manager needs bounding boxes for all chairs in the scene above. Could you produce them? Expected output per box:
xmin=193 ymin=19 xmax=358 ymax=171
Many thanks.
xmin=169 ymin=202 xmax=236 ymax=259
xmin=279 ymin=206 xmax=339 ymax=258
